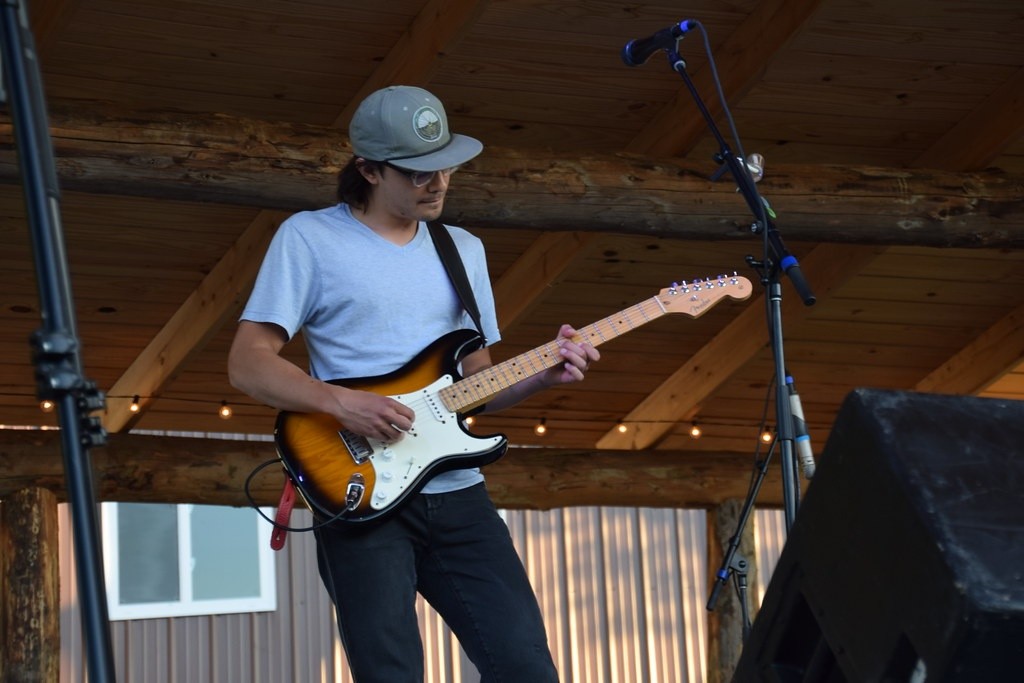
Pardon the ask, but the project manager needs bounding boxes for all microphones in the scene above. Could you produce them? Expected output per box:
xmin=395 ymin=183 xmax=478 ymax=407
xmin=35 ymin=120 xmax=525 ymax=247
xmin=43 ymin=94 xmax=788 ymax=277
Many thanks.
xmin=621 ymin=18 xmax=700 ymax=69
xmin=784 ymin=369 xmax=816 ymax=480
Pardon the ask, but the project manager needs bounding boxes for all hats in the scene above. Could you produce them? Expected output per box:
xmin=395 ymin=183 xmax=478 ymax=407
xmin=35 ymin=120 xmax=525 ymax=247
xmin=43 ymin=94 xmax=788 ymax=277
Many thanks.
xmin=348 ymin=85 xmax=484 ymax=172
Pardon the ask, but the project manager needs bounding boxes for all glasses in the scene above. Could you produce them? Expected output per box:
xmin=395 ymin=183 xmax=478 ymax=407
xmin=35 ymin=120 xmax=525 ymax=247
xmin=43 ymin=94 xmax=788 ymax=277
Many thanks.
xmin=375 ymin=160 xmax=459 ymax=187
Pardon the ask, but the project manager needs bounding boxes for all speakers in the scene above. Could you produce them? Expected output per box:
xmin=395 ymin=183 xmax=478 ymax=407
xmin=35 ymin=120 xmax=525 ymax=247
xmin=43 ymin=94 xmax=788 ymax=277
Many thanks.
xmin=725 ymin=387 xmax=1023 ymax=683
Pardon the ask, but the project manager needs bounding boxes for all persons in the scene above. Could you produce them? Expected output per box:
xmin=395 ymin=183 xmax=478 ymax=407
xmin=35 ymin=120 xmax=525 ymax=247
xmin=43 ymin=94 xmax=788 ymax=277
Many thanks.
xmin=227 ymin=83 xmax=600 ymax=683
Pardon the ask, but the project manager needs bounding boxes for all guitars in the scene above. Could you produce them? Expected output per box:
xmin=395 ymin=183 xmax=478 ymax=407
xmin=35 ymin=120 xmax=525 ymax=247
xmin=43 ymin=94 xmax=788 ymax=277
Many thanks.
xmin=273 ymin=268 xmax=753 ymax=534
